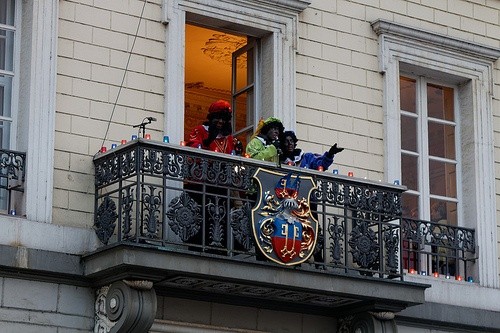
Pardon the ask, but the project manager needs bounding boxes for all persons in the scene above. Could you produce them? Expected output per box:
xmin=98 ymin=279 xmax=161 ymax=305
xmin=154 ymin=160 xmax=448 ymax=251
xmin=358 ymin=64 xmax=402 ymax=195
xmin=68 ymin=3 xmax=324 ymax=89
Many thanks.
xmin=188 ymin=99 xmax=242 ymax=260
xmin=247 ymin=117 xmax=285 ymax=266
xmin=280 ymin=131 xmax=345 ymax=269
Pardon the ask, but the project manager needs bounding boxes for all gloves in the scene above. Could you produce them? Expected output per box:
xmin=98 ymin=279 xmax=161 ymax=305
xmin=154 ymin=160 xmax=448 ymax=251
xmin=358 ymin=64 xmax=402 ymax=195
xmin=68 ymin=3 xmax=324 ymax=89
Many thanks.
xmin=274 ymin=139 xmax=281 ymax=148
xmin=329 ymin=143 xmax=344 ymax=155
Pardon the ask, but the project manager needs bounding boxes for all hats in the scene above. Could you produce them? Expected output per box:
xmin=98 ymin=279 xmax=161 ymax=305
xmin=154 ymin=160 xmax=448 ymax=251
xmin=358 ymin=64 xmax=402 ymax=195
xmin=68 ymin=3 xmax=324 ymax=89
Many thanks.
xmin=257 ymin=117 xmax=280 ymax=134
xmin=208 ymin=101 xmax=232 ymax=113
